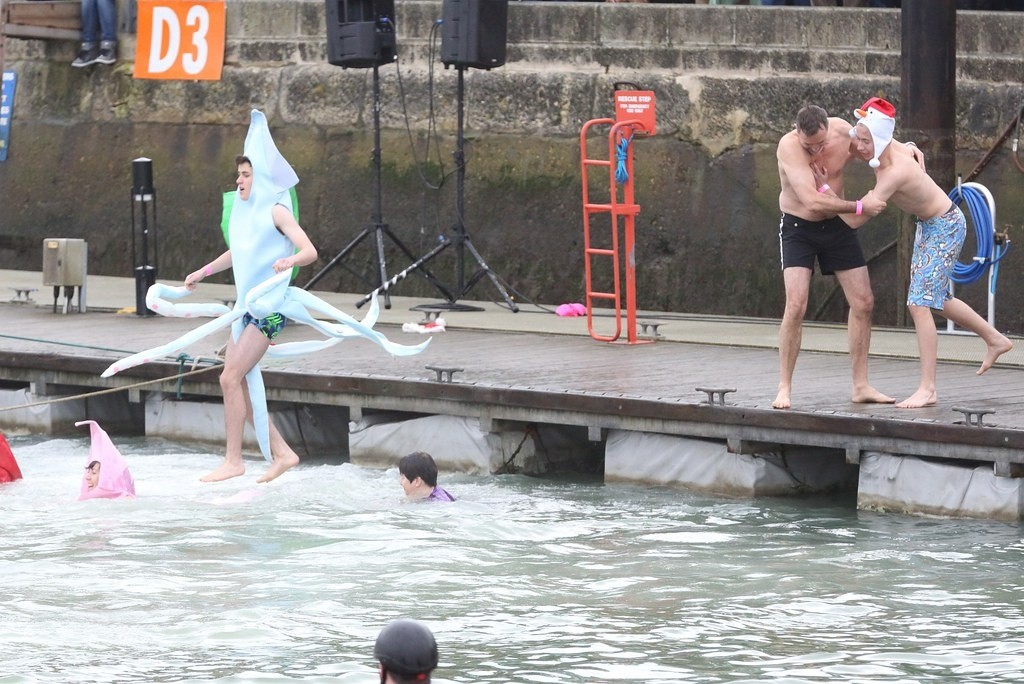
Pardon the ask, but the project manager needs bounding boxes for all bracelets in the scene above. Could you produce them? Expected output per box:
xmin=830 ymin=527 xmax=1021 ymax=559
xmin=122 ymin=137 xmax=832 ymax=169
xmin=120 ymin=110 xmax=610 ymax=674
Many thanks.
xmin=904 ymin=141 xmax=916 ymax=147
xmin=205 ymin=265 xmax=211 ymax=276
xmin=818 ymin=184 xmax=830 ymax=193
xmin=855 ymin=199 xmax=862 ymax=216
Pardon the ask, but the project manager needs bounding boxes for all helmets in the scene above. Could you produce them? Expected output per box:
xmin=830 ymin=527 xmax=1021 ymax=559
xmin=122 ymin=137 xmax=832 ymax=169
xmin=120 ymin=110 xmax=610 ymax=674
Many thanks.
xmin=375 ymin=620 xmax=437 ymax=675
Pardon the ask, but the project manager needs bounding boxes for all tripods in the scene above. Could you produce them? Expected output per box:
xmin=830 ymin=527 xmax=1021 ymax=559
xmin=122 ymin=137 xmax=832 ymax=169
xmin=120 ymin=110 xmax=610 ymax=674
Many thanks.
xmin=303 ymin=66 xmax=455 ymax=308
xmin=355 ymin=69 xmax=518 ymax=313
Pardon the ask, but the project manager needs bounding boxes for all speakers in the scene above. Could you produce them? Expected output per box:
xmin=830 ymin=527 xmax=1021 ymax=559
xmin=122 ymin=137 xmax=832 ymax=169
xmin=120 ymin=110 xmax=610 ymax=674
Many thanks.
xmin=326 ymin=0 xmax=398 ymax=68
xmin=441 ymin=0 xmax=508 ymax=70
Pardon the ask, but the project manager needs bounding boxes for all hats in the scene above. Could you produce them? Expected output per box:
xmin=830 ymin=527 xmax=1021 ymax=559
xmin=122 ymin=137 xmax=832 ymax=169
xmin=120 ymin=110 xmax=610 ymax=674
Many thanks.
xmin=849 ymin=97 xmax=896 ymax=168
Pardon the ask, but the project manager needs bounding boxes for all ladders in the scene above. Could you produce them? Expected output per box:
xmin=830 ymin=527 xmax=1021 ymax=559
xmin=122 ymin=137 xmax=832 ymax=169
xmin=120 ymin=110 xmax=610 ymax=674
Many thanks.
xmin=580 ymin=117 xmax=653 ymax=345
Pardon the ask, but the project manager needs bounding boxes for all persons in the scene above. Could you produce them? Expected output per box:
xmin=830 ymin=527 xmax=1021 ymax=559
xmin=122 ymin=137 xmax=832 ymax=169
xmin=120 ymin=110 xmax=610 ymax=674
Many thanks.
xmin=75 ymin=418 xmax=134 ymax=503
xmin=772 ymin=104 xmax=927 ymax=409
xmin=399 ymin=450 xmax=456 ymax=503
xmin=185 ymin=157 xmax=320 ymax=483
xmin=71 ymin=0 xmax=117 ymax=68
xmin=374 ymin=619 xmax=438 ymax=684
xmin=812 ymin=96 xmax=1012 ymax=408
